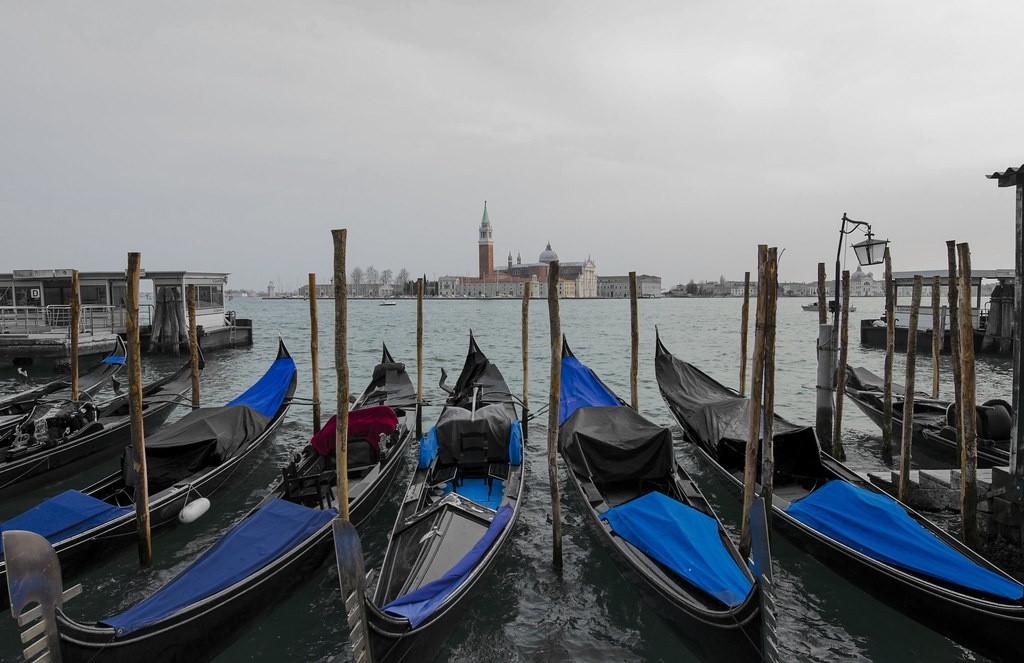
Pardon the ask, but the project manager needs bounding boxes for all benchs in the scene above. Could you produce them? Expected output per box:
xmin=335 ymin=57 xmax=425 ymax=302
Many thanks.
xmin=429 ymin=455 xmax=460 ymax=493
xmin=488 ymin=454 xmax=510 ymax=496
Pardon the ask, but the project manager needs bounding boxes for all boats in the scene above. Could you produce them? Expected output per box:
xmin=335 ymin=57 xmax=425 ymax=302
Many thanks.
xmin=380 ymin=301 xmax=396 ymax=306
xmin=55 ymin=338 xmax=418 ymax=648
xmin=0 ymin=325 xmax=299 ymax=576
xmin=802 ymin=302 xmax=856 ymax=313
xmin=844 ymin=367 xmax=1011 ymax=467
xmin=557 ymin=333 xmax=759 ymax=632
xmin=0 ymin=335 xmax=206 ymax=490
xmin=654 ymin=324 xmax=1024 ymax=623
xmin=363 ymin=330 xmax=525 ymax=639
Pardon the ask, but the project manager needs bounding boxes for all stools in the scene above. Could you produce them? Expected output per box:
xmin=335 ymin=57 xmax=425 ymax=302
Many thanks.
xmin=308 ymin=472 xmax=336 ymax=500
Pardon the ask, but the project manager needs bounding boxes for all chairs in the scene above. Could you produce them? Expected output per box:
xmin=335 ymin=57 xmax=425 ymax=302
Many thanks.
xmin=281 ymin=462 xmax=336 ymax=511
xmin=946 ymin=398 xmax=1014 ymax=453
xmin=458 ymin=431 xmax=490 ymax=486
